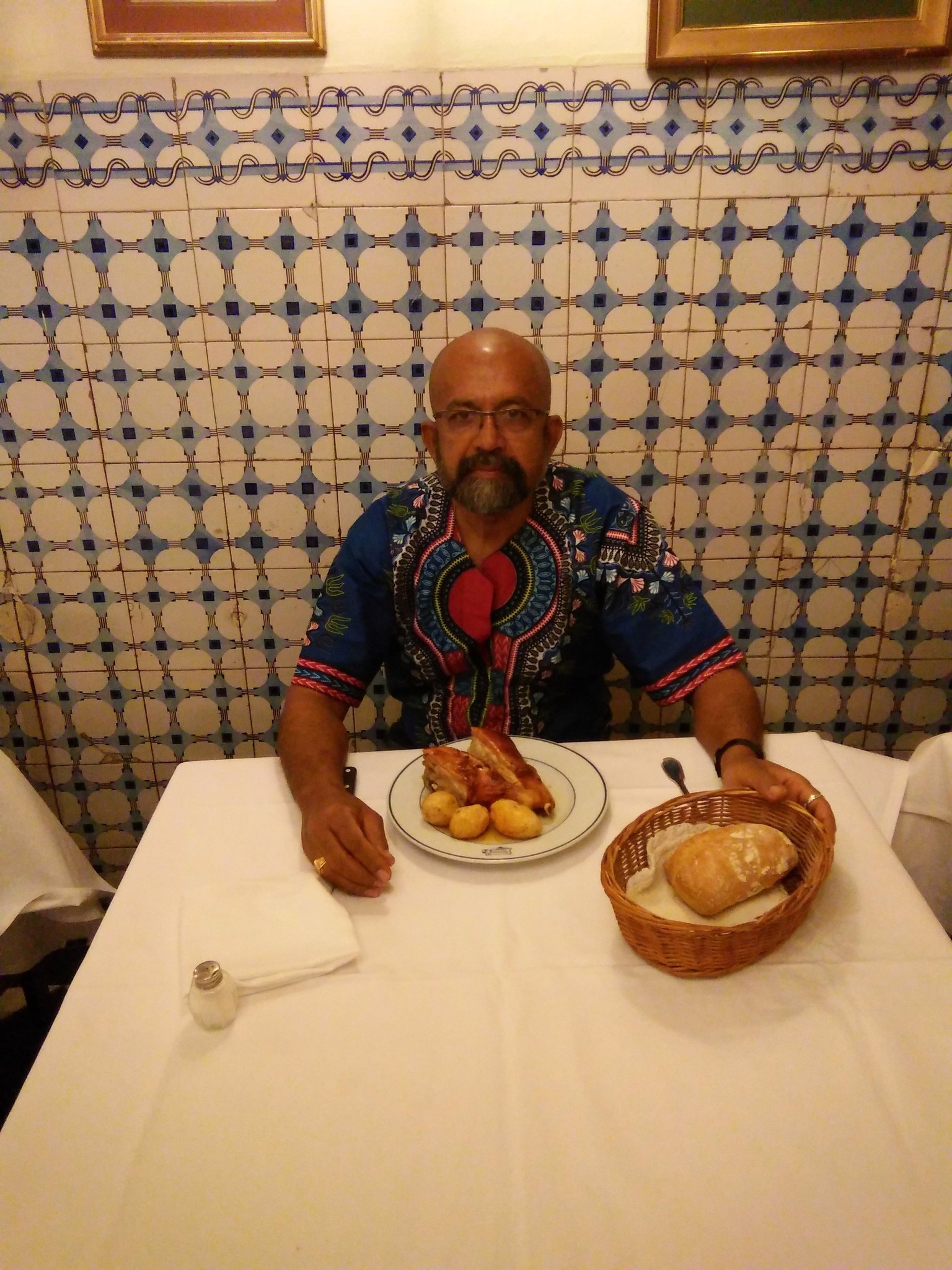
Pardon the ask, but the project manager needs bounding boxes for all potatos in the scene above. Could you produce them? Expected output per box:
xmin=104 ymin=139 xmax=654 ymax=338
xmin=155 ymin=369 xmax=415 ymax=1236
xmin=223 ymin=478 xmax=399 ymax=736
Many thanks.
xmin=449 ymin=804 xmax=490 ymax=840
xmin=489 ymin=799 xmax=543 ymax=841
xmin=421 ymin=790 xmax=458 ymax=827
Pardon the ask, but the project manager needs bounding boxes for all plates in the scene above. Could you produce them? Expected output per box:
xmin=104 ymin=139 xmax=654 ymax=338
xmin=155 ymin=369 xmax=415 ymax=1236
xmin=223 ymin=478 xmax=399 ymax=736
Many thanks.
xmin=388 ymin=736 xmax=607 ymax=865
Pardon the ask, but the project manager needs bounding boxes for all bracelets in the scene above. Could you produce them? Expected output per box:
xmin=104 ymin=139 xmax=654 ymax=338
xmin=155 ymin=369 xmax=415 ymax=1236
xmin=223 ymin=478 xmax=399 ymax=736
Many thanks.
xmin=713 ymin=738 xmax=765 ymax=778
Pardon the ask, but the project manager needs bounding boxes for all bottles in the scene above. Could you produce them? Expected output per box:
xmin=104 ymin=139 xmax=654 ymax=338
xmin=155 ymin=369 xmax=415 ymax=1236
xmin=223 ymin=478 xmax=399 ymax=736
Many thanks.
xmin=187 ymin=961 xmax=240 ymax=1030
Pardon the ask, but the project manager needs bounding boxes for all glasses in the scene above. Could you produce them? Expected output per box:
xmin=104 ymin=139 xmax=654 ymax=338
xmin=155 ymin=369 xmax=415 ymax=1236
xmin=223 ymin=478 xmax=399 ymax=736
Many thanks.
xmin=432 ymin=408 xmax=548 ymax=441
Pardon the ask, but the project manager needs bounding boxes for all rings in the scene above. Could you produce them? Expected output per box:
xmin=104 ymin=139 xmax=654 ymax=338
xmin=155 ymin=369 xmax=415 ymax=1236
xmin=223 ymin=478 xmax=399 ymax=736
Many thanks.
xmin=313 ymin=857 xmax=326 ymax=876
xmin=804 ymin=792 xmax=827 ymax=809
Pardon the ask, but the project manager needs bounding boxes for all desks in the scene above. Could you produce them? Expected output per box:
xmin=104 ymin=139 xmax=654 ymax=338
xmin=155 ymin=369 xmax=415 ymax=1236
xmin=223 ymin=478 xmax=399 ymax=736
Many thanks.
xmin=0 ymin=729 xmax=952 ymax=1270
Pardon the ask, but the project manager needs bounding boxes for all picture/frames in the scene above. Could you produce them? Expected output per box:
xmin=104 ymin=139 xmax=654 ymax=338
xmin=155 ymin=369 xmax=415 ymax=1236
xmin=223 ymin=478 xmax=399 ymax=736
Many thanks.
xmin=85 ymin=0 xmax=328 ymax=58
xmin=643 ymin=0 xmax=952 ymax=72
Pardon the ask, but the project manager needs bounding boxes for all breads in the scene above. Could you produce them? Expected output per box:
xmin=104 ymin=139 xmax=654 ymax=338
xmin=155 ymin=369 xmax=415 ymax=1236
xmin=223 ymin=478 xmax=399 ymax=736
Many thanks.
xmin=665 ymin=823 xmax=799 ymax=916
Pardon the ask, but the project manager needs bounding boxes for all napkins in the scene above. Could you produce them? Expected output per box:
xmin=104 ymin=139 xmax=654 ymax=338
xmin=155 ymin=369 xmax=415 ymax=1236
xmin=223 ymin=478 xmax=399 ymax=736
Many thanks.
xmin=180 ymin=871 xmax=362 ymax=999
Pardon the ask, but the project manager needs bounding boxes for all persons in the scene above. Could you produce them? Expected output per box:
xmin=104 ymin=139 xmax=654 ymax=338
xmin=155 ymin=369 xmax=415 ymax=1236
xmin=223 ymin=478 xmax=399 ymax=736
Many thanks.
xmin=275 ymin=328 xmax=836 ymax=898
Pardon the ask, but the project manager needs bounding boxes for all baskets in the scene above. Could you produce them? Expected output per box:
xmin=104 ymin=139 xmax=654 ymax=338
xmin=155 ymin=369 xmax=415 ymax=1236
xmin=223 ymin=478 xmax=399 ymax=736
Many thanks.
xmin=600 ymin=787 xmax=833 ymax=981
